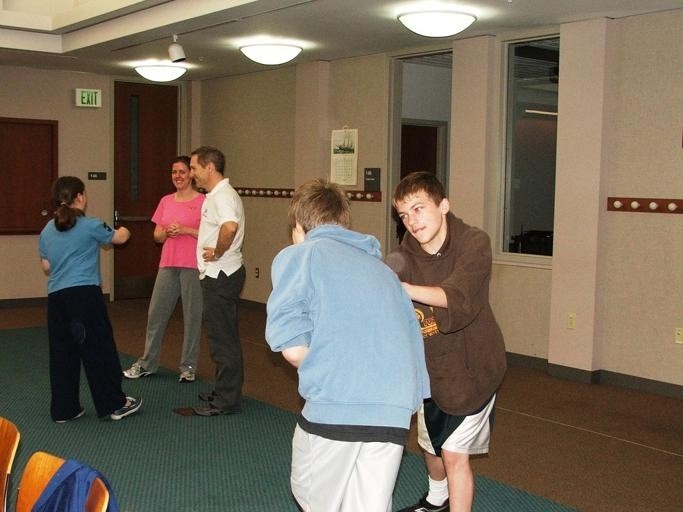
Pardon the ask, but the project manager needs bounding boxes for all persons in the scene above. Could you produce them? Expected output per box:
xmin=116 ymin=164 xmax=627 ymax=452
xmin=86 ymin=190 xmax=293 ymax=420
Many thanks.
xmin=38 ymin=176 xmax=142 ymax=423
xmin=380 ymin=170 xmax=507 ymax=512
xmin=122 ymin=155 xmax=206 ymax=383
xmin=189 ymin=146 xmax=246 ymax=415
xmin=265 ymin=177 xmax=431 ymax=512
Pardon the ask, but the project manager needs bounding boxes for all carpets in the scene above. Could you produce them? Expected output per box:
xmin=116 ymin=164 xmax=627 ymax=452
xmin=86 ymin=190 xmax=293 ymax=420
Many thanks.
xmin=1 ymin=315 xmax=580 ymax=512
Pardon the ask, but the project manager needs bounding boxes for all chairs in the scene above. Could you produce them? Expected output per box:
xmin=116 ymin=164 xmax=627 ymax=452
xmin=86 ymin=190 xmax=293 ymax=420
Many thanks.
xmin=1 ymin=416 xmax=19 ymax=512
xmin=17 ymin=450 xmax=110 ymax=512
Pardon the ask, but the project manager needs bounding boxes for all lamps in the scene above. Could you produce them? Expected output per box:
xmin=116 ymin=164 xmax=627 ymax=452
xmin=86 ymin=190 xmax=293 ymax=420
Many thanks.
xmin=169 ymin=34 xmax=189 ymax=63
xmin=398 ymin=10 xmax=479 ymax=42
xmin=240 ymin=43 xmax=303 ymax=67
xmin=134 ymin=60 xmax=188 ymax=83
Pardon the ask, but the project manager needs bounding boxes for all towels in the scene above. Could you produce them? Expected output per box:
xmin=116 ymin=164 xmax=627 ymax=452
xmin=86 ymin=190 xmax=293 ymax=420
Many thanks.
xmin=31 ymin=459 xmax=119 ymax=512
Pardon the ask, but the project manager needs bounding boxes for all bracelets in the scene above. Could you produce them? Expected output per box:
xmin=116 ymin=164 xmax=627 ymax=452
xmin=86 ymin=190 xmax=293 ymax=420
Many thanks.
xmin=211 ymin=250 xmax=218 ymax=260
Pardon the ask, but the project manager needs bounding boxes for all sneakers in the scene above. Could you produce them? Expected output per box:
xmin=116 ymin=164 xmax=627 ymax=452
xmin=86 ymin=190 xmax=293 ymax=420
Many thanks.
xmin=122 ymin=361 xmax=154 ymax=379
xmin=178 ymin=372 xmax=195 ymax=383
xmin=194 ymin=404 xmax=242 ymax=416
xmin=393 ymin=495 xmax=450 ymax=511
xmin=199 ymin=390 xmax=220 ymax=399
xmin=111 ymin=396 xmax=142 ymax=419
xmin=55 ymin=404 xmax=87 ymax=423
